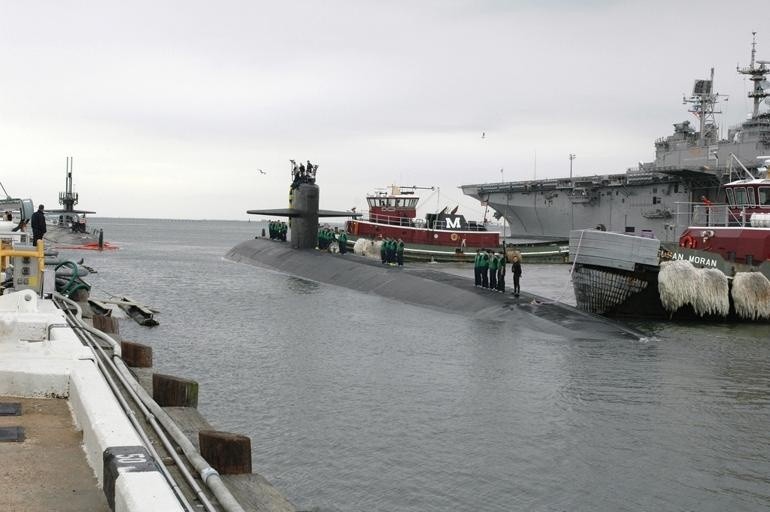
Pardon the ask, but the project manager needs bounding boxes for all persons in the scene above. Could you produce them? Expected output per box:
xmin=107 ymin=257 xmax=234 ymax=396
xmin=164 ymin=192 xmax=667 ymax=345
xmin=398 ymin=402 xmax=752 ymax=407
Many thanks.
xmin=473 ymin=248 xmax=507 ymax=293
xmin=31 ymin=204 xmax=47 ymax=246
xmin=267 ymin=219 xmax=288 ymax=242
xmin=701 ymin=196 xmax=713 ymax=213
xmin=380 ymin=236 xmax=405 ymax=267
xmin=293 ymin=159 xmax=318 ymax=184
xmin=72 ymin=215 xmax=87 ymax=234
xmin=318 ymin=228 xmax=348 ymax=256
xmin=2 ymin=210 xmax=12 ymax=221
xmin=511 ymin=255 xmax=522 ymax=297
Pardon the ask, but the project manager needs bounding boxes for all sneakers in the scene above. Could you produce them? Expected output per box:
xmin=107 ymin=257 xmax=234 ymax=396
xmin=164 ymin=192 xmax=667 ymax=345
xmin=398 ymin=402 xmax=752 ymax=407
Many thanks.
xmin=512 ymin=291 xmax=519 ymax=296
xmin=473 ymin=284 xmax=488 ymax=289
xmin=489 ymin=288 xmax=504 ymax=293
